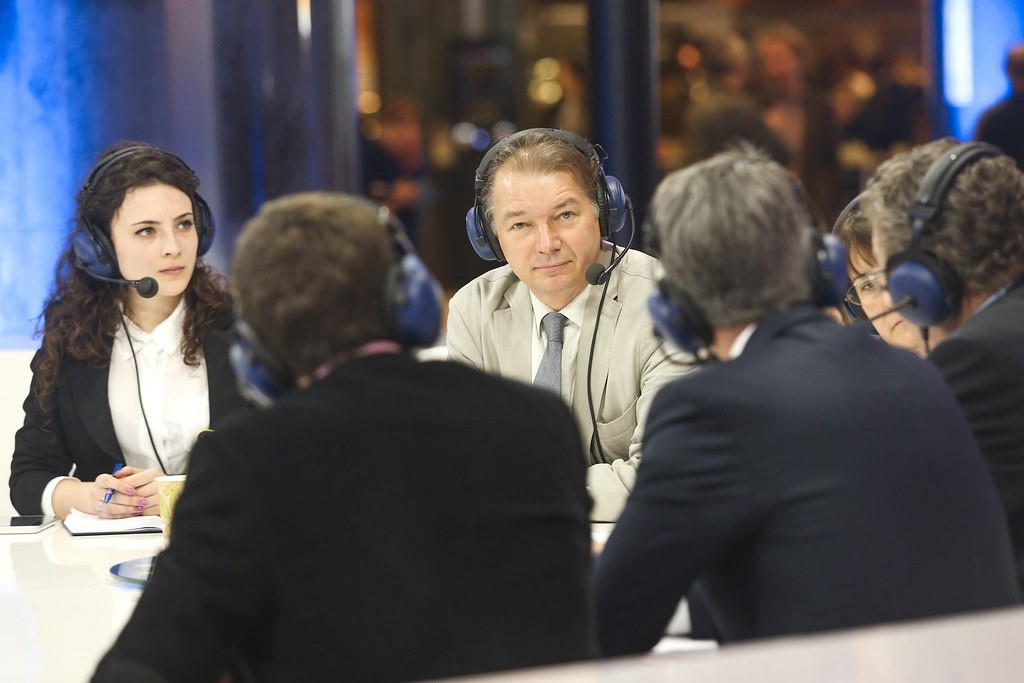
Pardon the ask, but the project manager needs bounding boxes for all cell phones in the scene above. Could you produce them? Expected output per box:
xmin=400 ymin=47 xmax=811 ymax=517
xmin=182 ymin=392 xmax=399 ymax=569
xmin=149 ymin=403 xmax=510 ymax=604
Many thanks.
xmin=0 ymin=515 xmax=60 ymax=534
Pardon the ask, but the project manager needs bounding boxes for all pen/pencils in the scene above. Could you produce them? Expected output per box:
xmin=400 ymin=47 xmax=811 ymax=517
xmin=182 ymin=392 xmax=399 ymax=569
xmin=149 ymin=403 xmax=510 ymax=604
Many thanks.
xmin=105 ymin=463 xmax=123 ymax=504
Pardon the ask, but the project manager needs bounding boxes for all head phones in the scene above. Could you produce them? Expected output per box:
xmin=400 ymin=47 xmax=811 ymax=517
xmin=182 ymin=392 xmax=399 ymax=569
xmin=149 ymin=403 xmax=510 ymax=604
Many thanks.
xmin=466 ymin=127 xmax=628 ymax=262
xmin=887 ymin=141 xmax=1001 ymax=327
xmin=227 ymin=207 xmax=445 ymax=408
xmin=646 ymin=170 xmax=848 ymax=352
xmin=73 ymin=146 xmax=216 ymax=277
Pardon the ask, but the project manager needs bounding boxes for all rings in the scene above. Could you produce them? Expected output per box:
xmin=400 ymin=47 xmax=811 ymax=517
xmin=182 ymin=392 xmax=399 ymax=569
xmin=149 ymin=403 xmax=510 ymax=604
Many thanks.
xmin=99 ymin=501 xmax=103 ymax=512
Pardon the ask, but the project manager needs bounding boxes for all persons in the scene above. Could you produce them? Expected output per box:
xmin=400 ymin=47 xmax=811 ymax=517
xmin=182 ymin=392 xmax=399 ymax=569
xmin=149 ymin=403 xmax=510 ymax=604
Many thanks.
xmin=682 ymin=19 xmax=942 ymax=197
xmin=598 ymin=153 xmax=1021 ymax=654
xmin=839 ymin=138 xmax=1024 ymax=582
xmin=448 ymin=131 xmax=706 ymax=523
xmin=90 ymin=193 xmax=596 ymax=683
xmin=8 ymin=142 xmax=254 ymax=518
xmin=975 ymin=46 xmax=1024 ymax=173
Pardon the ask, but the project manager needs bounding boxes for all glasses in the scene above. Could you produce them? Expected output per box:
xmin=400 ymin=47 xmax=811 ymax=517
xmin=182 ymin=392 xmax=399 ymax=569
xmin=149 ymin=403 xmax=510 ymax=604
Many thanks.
xmin=845 ymin=278 xmax=884 ymax=306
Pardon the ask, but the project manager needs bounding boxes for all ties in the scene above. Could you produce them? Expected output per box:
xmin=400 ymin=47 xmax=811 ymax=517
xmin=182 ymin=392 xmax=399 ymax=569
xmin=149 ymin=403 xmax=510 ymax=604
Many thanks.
xmin=533 ymin=312 xmax=569 ymax=398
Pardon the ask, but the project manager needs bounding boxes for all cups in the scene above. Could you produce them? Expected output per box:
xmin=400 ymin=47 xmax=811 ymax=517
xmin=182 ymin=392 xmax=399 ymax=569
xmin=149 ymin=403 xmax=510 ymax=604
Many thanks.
xmin=155 ymin=475 xmax=186 ymax=551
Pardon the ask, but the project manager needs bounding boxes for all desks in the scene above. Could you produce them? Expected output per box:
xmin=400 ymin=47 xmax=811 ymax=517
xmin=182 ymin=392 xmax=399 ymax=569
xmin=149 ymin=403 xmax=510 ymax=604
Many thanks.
xmin=0 ymin=505 xmax=691 ymax=683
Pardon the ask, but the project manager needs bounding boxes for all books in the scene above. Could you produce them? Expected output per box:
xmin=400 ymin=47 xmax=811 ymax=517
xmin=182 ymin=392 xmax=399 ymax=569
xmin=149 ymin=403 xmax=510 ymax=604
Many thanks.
xmin=62 ymin=508 xmax=163 ymax=536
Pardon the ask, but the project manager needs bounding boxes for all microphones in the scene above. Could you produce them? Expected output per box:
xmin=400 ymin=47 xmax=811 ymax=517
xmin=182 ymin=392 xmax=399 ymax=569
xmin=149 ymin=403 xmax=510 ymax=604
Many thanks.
xmin=585 ymin=206 xmax=635 ymax=285
xmin=81 ymin=267 xmax=159 ymax=298
